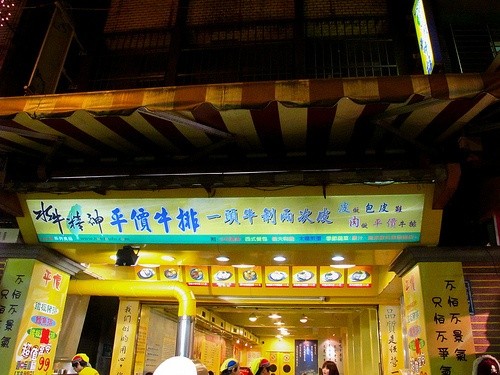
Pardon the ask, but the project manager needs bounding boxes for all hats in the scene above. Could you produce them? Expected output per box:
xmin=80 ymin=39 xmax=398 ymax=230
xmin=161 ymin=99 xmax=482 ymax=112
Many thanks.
xmin=472 ymin=355 xmax=500 ymax=375
xmin=71 ymin=353 xmax=89 ymax=367
xmin=220 ymin=358 xmax=239 ymax=372
xmin=249 ymin=358 xmax=271 ymax=375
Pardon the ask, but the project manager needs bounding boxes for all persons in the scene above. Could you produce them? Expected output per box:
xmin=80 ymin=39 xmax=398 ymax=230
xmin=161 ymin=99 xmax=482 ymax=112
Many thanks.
xmin=321 ymin=361 xmax=339 ymax=375
xmin=71 ymin=355 xmax=100 ymax=375
xmin=208 ymin=359 xmax=271 ymax=375
